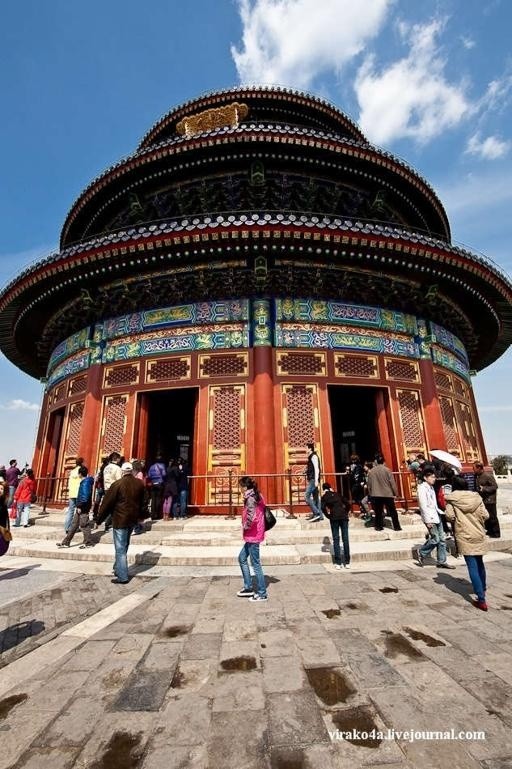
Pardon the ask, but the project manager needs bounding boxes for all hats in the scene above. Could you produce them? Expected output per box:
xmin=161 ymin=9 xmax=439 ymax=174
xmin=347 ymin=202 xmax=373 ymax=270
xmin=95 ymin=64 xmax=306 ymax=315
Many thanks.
xmin=121 ymin=462 xmax=134 ymax=472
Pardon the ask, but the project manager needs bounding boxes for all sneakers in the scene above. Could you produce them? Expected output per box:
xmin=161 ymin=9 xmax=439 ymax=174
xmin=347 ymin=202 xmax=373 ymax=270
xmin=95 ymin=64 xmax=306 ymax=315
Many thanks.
xmin=415 ymin=548 xmax=424 ymax=566
xmin=237 ymin=587 xmax=254 ymax=596
xmin=163 ymin=517 xmax=188 ymax=521
xmin=309 ymin=514 xmax=321 ymax=523
xmin=335 ymin=562 xmax=350 ymax=569
xmin=436 ymin=563 xmax=456 ymax=569
xmin=251 ymin=593 xmax=269 ymax=602
xmin=111 ymin=579 xmax=128 ymax=584
xmin=474 ymin=601 xmax=487 ymax=611
xmin=78 ymin=544 xmax=93 ymax=549
xmin=56 ymin=542 xmax=69 ymax=547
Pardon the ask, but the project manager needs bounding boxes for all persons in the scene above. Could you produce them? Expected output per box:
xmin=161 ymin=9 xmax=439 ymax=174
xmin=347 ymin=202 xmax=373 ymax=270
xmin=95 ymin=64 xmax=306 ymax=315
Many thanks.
xmin=237 ymin=476 xmax=268 ymax=601
xmin=321 ymin=483 xmax=351 ymax=569
xmin=345 ymin=449 xmax=500 ymax=569
xmin=304 ymin=443 xmax=324 ymax=521
xmin=444 ymin=475 xmax=490 ymax=611
xmin=0 ymin=452 xmax=189 ymax=584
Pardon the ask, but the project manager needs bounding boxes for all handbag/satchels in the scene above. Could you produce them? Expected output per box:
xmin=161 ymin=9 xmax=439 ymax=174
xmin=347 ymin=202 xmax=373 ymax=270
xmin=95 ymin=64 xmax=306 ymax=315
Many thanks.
xmin=265 ymin=507 xmax=277 ymax=531
xmin=29 ymin=492 xmax=36 ymax=503
